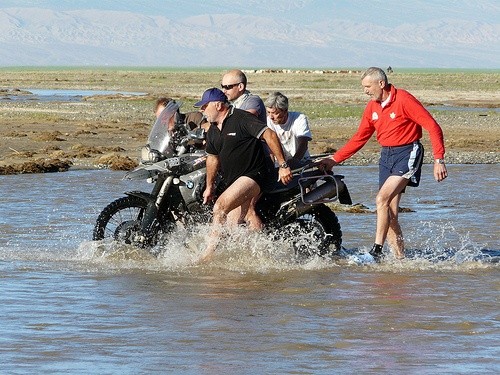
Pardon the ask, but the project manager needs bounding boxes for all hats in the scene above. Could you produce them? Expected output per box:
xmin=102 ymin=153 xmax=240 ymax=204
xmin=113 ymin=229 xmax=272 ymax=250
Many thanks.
xmin=194 ymin=88 xmax=228 ymax=107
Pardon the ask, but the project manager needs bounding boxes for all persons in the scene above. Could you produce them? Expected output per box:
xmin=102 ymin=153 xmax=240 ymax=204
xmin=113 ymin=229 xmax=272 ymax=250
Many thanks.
xmin=199 ymin=70 xmax=267 ymax=233
xmin=194 ymin=87 xmax=293 ymax=263
xmin=157 ymin=98 xmax=206 ymax=147
xmin=264 ymin=91 xmax=313 ymax=163
xmin=318 ymin=68 xmax=448 ymax=258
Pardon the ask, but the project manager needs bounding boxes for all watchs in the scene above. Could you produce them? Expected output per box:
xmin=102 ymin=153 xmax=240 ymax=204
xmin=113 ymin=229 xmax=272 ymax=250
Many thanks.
xmin=281 ymin=162 xmax=288 ymax=168
xmin=435 ymin=159 xmax=444 ymax=163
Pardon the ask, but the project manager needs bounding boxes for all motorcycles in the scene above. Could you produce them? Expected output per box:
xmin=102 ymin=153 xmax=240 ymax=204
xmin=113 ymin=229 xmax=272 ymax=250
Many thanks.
xmin=92 ymin=100 xmax=352 ymax=259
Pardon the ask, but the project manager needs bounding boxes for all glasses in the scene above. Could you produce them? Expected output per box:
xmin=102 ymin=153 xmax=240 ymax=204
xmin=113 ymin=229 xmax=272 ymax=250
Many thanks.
xmin=221 ymin=82 xmax=242 ymax=90
